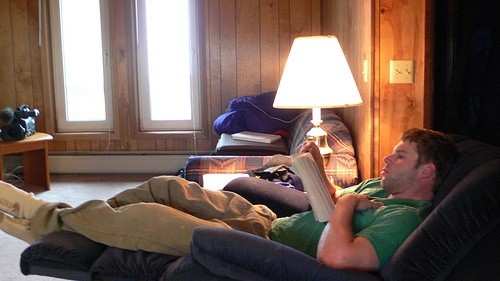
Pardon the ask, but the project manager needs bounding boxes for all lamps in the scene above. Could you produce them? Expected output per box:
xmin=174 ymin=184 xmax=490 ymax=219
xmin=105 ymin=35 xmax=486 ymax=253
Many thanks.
xmin=273 ymin=36 xmax=364 ymax=169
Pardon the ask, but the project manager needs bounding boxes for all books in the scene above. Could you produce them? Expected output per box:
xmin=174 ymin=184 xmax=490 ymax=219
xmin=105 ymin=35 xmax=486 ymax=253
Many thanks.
xmin=232 ymin=130 xmax=281 ymax=144
xmin=288 ymin=151 xmax=334 ymax=222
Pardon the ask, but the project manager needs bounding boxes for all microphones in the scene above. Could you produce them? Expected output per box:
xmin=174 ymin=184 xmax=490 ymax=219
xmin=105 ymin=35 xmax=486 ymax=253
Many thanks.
xmin=1 ymin=106 xmax=15 ymax=123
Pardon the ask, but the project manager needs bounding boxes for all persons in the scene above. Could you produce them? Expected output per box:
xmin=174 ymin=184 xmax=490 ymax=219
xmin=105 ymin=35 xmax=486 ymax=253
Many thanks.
xmin=0 ymin=128 xmax=459 ymax=272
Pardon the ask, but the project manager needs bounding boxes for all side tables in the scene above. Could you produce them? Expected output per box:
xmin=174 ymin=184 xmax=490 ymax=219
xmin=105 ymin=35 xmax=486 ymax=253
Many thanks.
xmin=0 ymin=132 xmax=53 ymax=190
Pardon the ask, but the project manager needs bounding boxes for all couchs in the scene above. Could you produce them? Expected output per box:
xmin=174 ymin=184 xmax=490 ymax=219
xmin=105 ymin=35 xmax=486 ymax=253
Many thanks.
xmin=19 ymin=135 xmax=500 ymax=281
xmin=176 ymin=110 xmax=358 ymax=188
